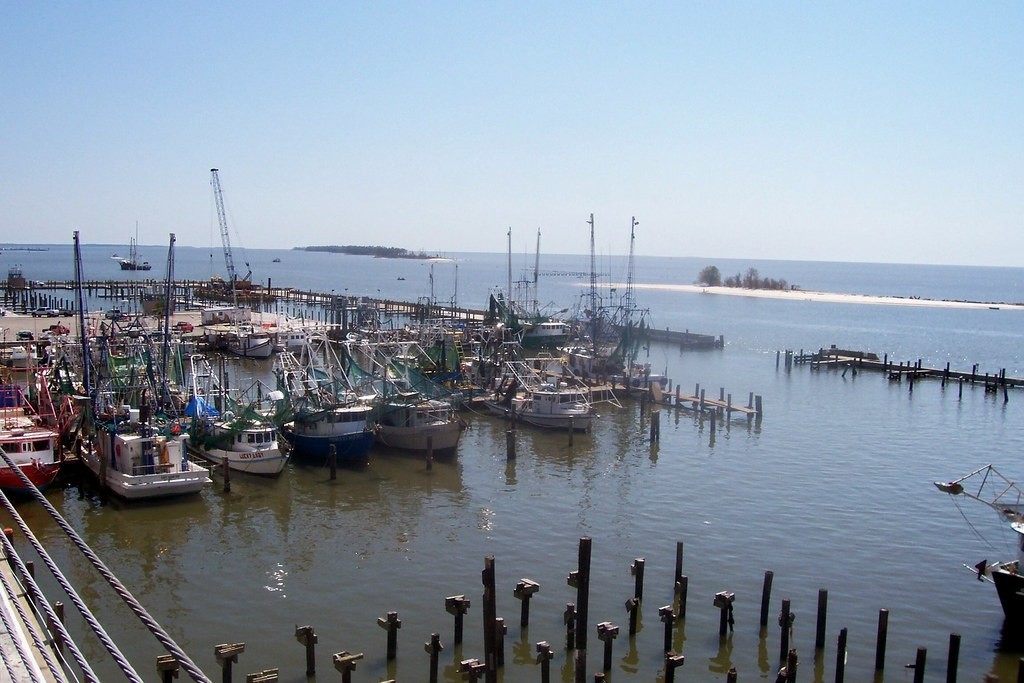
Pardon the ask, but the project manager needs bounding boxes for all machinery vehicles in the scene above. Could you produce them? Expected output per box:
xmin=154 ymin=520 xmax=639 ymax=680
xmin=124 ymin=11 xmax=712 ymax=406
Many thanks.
xmin=210 ymin=168 xmax=252 ymax=289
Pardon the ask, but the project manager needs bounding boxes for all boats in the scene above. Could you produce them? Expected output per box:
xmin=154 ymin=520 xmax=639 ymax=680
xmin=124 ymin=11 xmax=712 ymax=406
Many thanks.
xmin=111 ymin=254 xmax=152 ymax=271
xmin=553 ymin=212 xmax=670 ymax=396
xmin=0 ymin=213 xmax=622 ymax=501
xmin=934 ymin=465 xmax=1024 ymax=630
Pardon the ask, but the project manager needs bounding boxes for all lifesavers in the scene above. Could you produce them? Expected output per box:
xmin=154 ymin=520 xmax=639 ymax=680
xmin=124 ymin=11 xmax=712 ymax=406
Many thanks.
xmin=562 ymin=360 xmax=569 ymax=367
xmin=633 ymin=378 xmax=641 ymax=387
xmin=223 ymin=411 xmax=234 ymax=423
xmin=661 ymin=377 xmax=668 ymax=385
